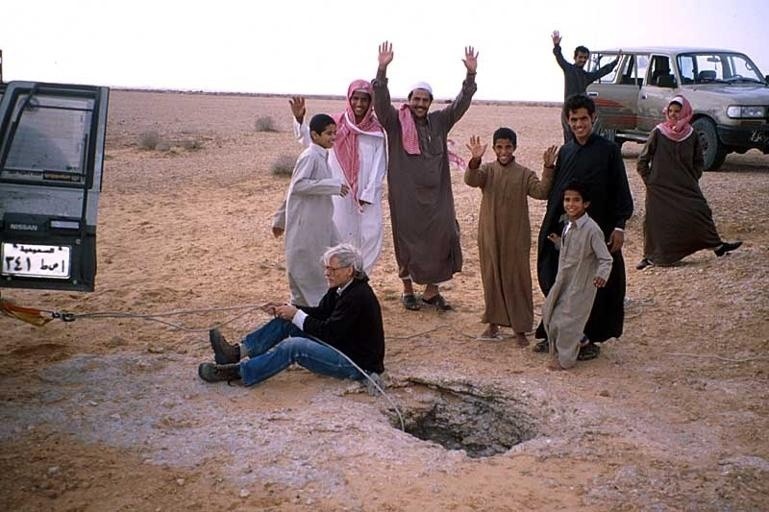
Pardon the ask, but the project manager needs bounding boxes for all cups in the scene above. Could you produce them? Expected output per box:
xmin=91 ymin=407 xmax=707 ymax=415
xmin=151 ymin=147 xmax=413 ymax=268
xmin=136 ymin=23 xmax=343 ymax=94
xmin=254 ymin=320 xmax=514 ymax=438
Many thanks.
xmin=326 ymin=266 xmax=349 ymax=277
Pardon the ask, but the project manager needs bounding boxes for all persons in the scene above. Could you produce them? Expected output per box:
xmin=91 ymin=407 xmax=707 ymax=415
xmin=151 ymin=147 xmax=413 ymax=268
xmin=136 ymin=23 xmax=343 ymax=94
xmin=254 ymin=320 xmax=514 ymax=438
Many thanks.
xmin=274 ymin=114 xmax=351 ymax=309
xmin=636 ymin=93 xmax=746 ymax=271
xmin=197 ymin=243 xmax=386 ymax=384
xmin=551 ymin=30 xmax=626 ymax=142
xmin=536 ymin=94 xmax=635 ymax=361
xmin=462 ymin=126 xmax=558 ymax=349
xmin=291 ymin=79 xmax=390 ymax=275
xmin=540 ymin=185 xmax=615 ymax=372
xmin=372 ymin=40 xmax=478 ymax=313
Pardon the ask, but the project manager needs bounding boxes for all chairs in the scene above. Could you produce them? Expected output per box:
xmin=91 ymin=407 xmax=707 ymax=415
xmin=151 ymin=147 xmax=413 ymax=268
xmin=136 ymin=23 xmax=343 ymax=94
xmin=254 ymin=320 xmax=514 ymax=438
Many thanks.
xmin=715 ymin=241 xmax=743 ymax=257
xmin=636 ymin=259 xmax=654 ymax=272
xmin=536 ymin=340 xmax=549 ymax=353
xmin=209 ymin=329 xmax=240 ymax=365
xmin=199 ymin=362 xmax=242 ymax=383
xmin=578 ymin=343 xmax=600 ymax=360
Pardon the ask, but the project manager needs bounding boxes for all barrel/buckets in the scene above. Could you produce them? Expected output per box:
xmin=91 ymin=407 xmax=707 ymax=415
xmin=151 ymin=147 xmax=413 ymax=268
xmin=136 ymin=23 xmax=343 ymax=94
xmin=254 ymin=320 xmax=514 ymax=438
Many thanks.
xmin=400 ymin=293 xmax=420 ymax=310
xmin=422 ymin=294 xmax=452 ymax=311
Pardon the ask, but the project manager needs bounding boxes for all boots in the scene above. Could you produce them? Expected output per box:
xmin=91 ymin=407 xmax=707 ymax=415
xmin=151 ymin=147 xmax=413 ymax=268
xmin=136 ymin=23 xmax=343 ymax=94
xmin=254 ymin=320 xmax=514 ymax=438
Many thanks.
xmin=0 ymin=50 xmax=111 ymax=292
xmin=584 ymin=47 xmax=769 ymax=172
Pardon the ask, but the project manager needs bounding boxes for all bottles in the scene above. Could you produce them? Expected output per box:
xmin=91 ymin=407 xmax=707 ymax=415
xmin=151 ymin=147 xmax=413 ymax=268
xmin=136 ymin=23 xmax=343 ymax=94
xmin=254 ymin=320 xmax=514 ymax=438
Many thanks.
xmin=410 ymin=81 xmax=432 ymax=94
xmin=671 ymin=97 xmax=683 ymax=107
